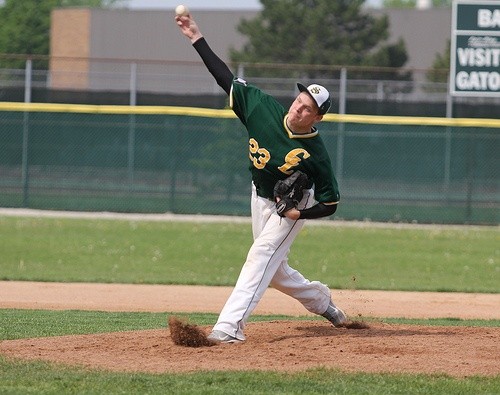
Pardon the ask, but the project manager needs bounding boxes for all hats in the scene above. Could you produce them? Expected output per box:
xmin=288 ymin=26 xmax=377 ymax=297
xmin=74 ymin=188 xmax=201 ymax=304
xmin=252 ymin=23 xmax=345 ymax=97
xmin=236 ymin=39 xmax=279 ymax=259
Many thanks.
xmin=297 ymin=83 xmax=332 ymax=115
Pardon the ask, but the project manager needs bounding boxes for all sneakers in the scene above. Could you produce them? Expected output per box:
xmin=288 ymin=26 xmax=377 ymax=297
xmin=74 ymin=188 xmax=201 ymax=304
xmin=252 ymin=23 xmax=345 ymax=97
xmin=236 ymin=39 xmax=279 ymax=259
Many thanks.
xmin=207 ymin=330 xmax=239 ymax=343
xmin=320 ymin=292 xmax=348 ymax=328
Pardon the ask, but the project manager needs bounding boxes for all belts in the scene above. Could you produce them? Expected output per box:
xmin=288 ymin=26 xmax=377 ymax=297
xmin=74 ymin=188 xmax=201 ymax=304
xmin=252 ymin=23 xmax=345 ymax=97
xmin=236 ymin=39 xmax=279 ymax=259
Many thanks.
xmin=254 ymin=181 xmax=260 ymax=194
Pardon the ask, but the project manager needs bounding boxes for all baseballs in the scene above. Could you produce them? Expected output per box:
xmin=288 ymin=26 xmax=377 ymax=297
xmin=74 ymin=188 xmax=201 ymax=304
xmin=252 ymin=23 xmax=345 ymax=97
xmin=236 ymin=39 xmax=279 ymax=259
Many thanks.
xmin=175 ymin=5 xmax=187 ymax=16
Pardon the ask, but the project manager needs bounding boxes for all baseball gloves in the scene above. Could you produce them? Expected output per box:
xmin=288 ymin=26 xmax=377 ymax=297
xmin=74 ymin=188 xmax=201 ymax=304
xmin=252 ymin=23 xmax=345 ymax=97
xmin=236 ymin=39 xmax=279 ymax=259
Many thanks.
xmin=273 ymin=170 xmax=314 ymax=218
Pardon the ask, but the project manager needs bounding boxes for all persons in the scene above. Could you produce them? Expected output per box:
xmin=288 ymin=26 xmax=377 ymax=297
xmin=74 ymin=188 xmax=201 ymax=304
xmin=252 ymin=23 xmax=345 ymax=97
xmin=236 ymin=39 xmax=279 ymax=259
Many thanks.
xmin=175 ymin=11 xmax=348 ymax=344
